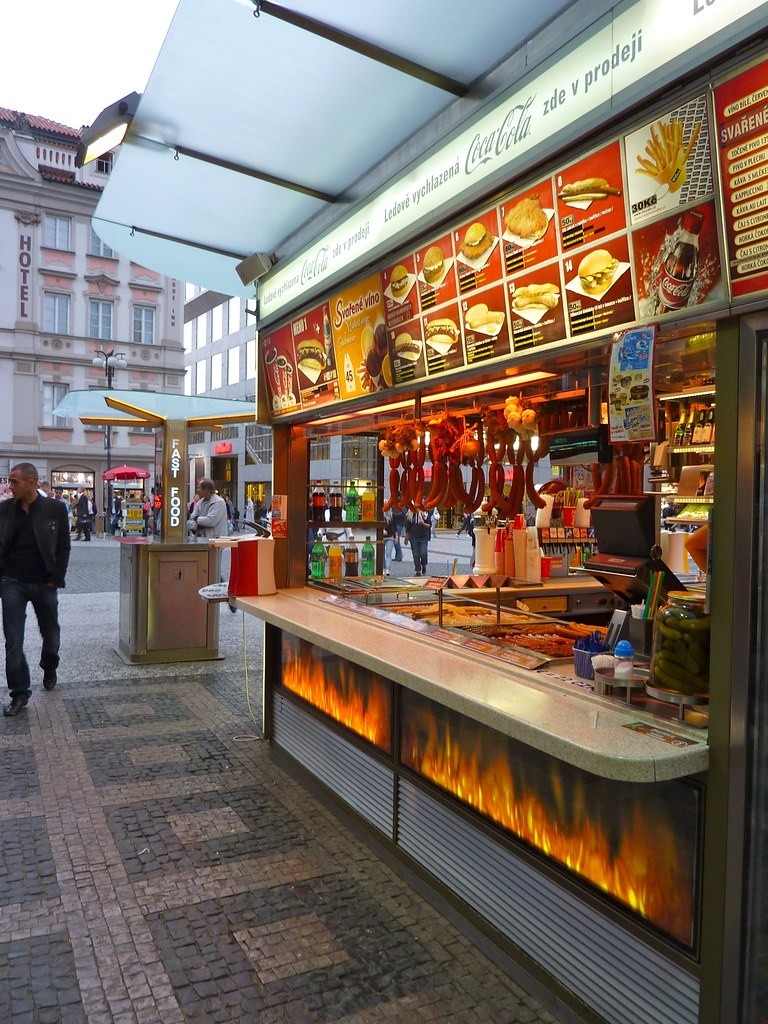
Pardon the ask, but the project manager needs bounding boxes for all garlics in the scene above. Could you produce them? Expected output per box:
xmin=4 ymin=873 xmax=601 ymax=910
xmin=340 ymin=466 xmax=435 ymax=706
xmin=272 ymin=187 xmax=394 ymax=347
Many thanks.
xmin=379 ymin=396 xmax=539 ymax=459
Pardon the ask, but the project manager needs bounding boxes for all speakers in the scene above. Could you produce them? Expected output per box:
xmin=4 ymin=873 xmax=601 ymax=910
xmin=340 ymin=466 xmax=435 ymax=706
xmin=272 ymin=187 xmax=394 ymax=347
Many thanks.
xmin=235 ymin=252 xmax=271 ymax=286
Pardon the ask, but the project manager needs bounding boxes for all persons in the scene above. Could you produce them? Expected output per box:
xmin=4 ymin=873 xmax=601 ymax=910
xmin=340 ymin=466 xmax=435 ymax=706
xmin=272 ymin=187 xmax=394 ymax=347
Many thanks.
xmin=37 ymin=481 xmax=151 ymax=542
xmin=662 ymin=502 xmax=675 ymax=531
xmin=383 ymin=494 xmax=440 ymax=576
xmin=0 ymin=462 xmax=71 ymax=716
xmin=188 ymin=476 xmax=272 ymax=613
xmin=455 ymin=508 xmax=477 ymax=567
xmin=308 ymin=491 xmax=355 ymax=579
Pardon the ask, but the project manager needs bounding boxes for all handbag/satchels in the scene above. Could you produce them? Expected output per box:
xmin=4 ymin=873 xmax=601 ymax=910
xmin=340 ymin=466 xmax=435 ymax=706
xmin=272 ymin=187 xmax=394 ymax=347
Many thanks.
xmin=79 ymin=517 xmax=92 ymax=527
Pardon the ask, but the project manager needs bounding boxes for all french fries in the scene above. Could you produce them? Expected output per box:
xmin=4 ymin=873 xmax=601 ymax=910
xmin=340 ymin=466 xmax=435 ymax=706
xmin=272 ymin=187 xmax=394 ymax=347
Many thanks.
xmin=635 ymin=122 xmax=702 ymax=177
xmin=357 ymin=359 xmax=377 ymax=393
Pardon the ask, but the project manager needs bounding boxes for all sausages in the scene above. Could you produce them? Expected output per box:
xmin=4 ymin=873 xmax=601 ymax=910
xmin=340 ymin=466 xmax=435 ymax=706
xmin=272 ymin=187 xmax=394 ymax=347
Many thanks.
xmin=555 ymin=622 xmax=608 ymax=639
xmin=392 ymin=343 xmax=420 ymax=355
xmin=532 ymin=404 xmax=587 ymax=463
xmin=583 ymin=443 xmax=644 ymax=510
xmin=382 ymin=411 xmax=546 ymax=518
xmin=469 ymin=312 xmax=503 ymax=329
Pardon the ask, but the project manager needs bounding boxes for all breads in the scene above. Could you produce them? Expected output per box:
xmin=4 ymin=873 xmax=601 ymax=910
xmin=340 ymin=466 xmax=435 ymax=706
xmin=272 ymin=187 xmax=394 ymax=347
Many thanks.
xmin=464 ymin=222 xmax=487 ymax=244
xmin=395 ymin=333 xmax=412 ymax=345
xmin=465 ymin=303 xmax=489 ymax=323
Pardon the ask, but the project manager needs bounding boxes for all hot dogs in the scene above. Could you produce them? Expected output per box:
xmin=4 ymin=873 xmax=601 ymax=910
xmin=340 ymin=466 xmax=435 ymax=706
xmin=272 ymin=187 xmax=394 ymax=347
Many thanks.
xmin=425 ymin=318 xmax=460 ymax=343
xmin=511 ymin=282 xmax=559 ymax=310
xmin=297 ymin=339 xmax=327 ymax=370
xmin=557 ymin=177 xmax=622 ymax=202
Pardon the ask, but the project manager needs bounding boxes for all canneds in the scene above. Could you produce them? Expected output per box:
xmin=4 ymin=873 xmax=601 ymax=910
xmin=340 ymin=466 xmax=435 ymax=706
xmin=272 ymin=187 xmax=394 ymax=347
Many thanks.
xmin=649 ymin=591 xmax=710 ymax=694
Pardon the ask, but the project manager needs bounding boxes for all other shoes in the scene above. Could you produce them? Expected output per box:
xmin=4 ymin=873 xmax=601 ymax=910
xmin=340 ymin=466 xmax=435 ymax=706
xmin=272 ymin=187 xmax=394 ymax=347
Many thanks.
xmin=72 ymin=538 xmax=79 ymax=541
xmin=456 ymin=533 xmax=460 ymax=538
xmin=431 ymin=536 xmax=437 ymax=539
xmin=422 ymin=566 xmax=427 ymax=575
xmin=81 ymin=539 xmax=91 ymax=542
xmin=391 ymin=558 xmax=402 ymax=562
xmin=382 ymin=568 xmax=390 ymax=577
xmin=415 ymin=570 xmax=421 ymax=576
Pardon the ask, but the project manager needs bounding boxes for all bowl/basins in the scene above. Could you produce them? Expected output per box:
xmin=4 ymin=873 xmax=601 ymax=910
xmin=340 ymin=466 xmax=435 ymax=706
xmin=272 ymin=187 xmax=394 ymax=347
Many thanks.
xmin=571 ymin=645 xmax=612 ymax=678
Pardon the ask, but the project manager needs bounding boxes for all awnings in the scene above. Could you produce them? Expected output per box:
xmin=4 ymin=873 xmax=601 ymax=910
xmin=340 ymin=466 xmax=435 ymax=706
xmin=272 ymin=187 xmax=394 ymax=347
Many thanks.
xmin=83 ymin=0 xmax=632 ymax=300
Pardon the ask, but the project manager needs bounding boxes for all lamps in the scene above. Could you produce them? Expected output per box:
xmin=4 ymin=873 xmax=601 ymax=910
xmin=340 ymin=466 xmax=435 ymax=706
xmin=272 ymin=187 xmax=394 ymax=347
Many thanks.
xmin=74 ymin=90 xmax=139 ymax=168
xmin=235 ymin=252 xmax=276 ymax=286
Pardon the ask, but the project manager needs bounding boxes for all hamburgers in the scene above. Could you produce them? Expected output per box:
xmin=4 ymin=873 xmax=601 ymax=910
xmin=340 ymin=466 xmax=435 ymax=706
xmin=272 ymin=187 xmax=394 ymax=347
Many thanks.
xmin=423 ymin=246 xmax=444 ymax=284
xmin=390 ymin=265 xmax=410 ymax=297
xmin=578 ymin=249 xmax=619 ymax=294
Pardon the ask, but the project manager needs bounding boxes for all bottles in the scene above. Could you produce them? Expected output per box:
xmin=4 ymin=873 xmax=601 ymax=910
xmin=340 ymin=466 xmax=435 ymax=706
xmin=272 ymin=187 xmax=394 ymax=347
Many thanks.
xmin=328 ymin=536 xmax=342 ymax=578
xmin=311 ymin=537 xmax=325 ymax=579
xmin=344 ymin=536 xmax=358 ymax=576
xmin=344 ymin=353 xmax=357 ymax=392
xmin=614 ymin=640 xmax=634 ymax=679
xmin=362 ymin=482 xmax=375 ymax=521
xmin=652 ymin=211 xmax=704 ymax=317
xmin=345 ymin=482 xmax=359 ymax=521
xmin=312 ymin=480 xmax=325 ymax=522
xmin=672 ymin=406 xmax=716 ymax=447
xmin=322 ymin=305 xmax=335 ymax=367
xmin=329 ymin=481 xmax=343 ymax=521
xmin=362 ymin=536 xmax=374 ymax=575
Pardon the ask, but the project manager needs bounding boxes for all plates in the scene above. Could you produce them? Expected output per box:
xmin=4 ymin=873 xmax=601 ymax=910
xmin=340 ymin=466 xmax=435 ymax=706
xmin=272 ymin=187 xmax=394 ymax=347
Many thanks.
xmin=596 ymin=651 xmax=703 ymax=705
xmin=298 ymin=198 xmax=632 ymax=385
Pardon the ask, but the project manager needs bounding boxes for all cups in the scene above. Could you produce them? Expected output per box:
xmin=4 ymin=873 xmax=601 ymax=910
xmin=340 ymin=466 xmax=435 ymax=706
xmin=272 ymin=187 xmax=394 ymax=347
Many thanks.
xmin=494 ymin=513 xmax=552 ymax=583
xmin=536 ymin=493 xmax=592 ymax=529
xmin=653 ymin=160 xmax=686 ymax=192
xmin=611 ymin=393 xmax=627 ymax=411
xmin=265 ymin=346 xmax=293 ymax=398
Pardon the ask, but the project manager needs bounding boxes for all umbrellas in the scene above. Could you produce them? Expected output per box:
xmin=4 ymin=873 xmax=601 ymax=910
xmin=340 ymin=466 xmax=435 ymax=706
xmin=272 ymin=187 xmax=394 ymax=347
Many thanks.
xmin=102 ymin=464 xmax=150 ymax=501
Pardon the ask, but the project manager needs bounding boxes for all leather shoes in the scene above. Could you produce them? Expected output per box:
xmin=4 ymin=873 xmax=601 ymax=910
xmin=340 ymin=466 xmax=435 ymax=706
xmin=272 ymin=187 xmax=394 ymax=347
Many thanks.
xmin=42 ymin=669 xmax=58 ymax=690
xmin=2 ymin=690 xmax=28 ymax=716
